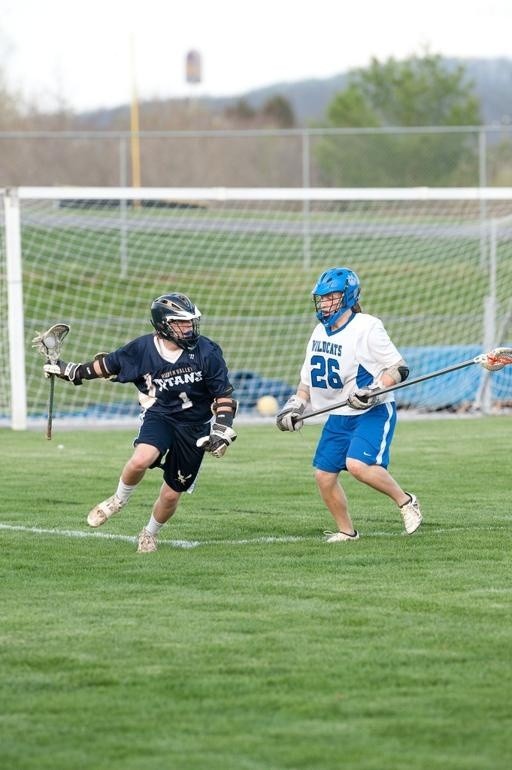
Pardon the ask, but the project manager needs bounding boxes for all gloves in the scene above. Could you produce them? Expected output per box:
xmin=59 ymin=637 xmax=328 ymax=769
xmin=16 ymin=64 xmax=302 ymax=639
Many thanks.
xmin=344 ymin=379 xmax=386 ymax=413
xmin=194 ymin=420 xmax=240 ymax=460
xmin=42 ymin=357 xmax=86 ymax=389
xmin=272 ymin=392 xmax=311 ymax=434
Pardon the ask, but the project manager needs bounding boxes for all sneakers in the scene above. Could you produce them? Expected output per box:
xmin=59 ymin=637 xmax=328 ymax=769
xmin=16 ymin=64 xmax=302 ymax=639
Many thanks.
xmin=320 ymin=527 xmax=362 ymax=548
xmin=86 ymin=492 xmax=131 ymax=530
xmin=135 ymin=524 xmax=160 ymax=555
xmin=398 ymin=488 xmax=427 ymax=537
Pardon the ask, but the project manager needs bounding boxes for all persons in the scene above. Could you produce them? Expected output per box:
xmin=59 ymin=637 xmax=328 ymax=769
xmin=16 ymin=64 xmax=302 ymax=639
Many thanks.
xmin=276 ymin=267 xmax=424 ymax=544
xmin=44 ymin=292 xmax=240 ymax=555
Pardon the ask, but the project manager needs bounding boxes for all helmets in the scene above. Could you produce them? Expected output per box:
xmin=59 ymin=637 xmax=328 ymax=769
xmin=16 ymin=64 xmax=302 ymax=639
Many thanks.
xmin=149 ymin=291 xmax=204 ymax=335
xmin=309 ymin=266 xmax=362 ymax=328
